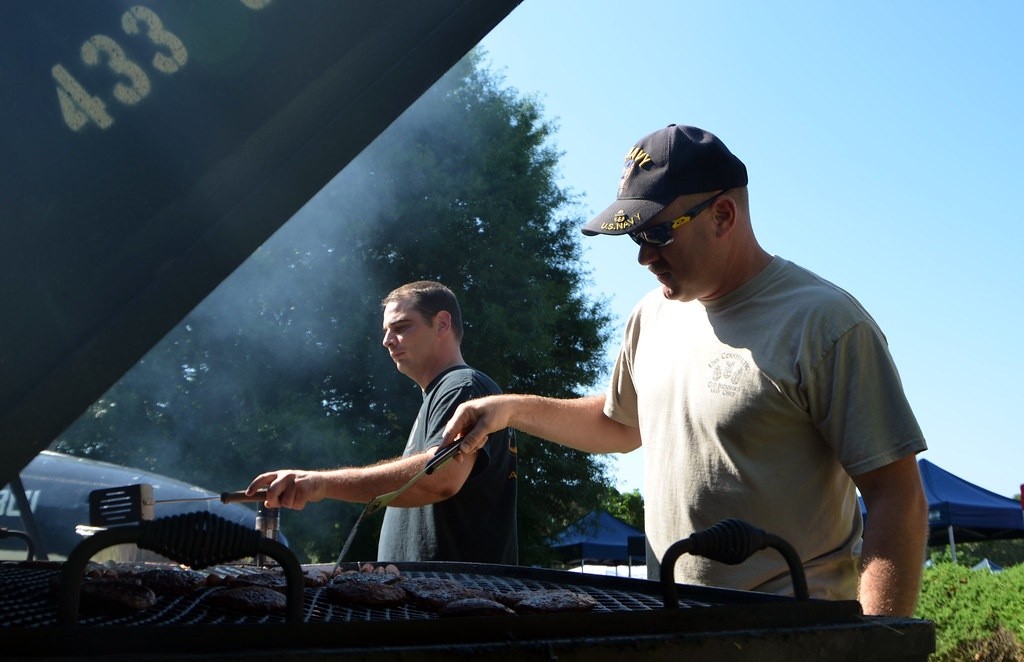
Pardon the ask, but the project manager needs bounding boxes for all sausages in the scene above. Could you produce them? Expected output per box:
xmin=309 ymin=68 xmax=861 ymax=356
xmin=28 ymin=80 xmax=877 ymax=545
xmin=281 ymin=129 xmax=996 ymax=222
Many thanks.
xmin=85 ymin=559 xmax=135 ymax=579
xmin=360 ymin=563 xmax=401 ymax=575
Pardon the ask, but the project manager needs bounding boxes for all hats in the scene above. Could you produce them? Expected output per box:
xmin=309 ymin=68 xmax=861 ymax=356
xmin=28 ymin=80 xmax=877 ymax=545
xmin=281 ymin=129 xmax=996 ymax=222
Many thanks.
xmin=581 ymin=124 xmax=748 ymax=237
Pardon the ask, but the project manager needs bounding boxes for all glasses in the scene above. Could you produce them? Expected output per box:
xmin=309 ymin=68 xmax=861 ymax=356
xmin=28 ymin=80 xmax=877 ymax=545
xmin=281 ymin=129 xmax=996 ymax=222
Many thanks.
xmin=630 ymin=190 xmax=730 ymax=247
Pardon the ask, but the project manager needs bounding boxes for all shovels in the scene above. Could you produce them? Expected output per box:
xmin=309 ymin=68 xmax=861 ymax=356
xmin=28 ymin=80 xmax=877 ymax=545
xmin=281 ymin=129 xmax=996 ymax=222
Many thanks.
xmin=87 ymin=482 xmax=270 ymax=527
xmin=327 ymin=439 xmax=464 ymax=577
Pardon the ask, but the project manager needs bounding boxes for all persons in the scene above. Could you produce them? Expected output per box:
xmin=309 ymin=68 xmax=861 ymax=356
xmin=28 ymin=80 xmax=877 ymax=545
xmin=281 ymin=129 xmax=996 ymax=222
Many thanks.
xmin=433 ymin=124 xmax=928 ymax=618
xmin=246 ymin=280 xmax=521 ymax=569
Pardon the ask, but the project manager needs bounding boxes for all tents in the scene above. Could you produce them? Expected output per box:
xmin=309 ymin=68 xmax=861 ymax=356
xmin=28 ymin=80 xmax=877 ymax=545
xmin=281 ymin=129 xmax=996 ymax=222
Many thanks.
xmin=857 ymin=459 xmax=1024 ymax=574
xmin=550 ymin=506 xmax=647 ymax=580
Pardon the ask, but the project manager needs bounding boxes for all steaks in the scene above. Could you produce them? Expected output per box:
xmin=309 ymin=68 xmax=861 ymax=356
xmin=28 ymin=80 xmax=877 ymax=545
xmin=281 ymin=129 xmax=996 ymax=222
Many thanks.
xmin=77 ymin=570 xmax=597 ymax=622
